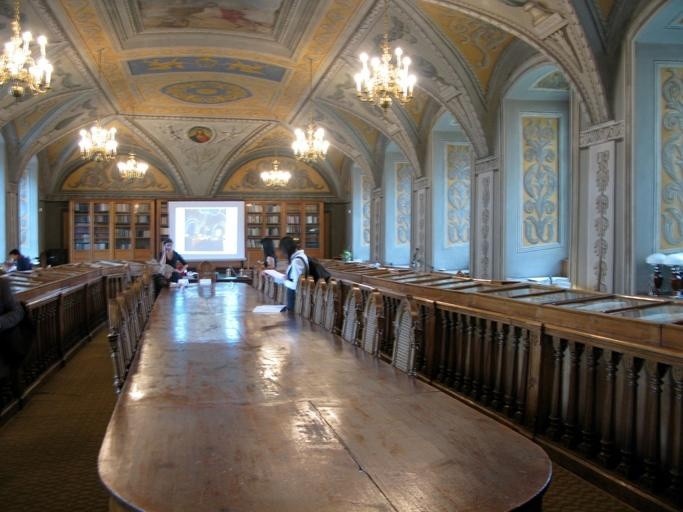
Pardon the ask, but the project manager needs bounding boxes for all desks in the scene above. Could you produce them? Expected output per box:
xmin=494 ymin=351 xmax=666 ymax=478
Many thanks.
xmin=94 ymin=279 xmax=552 ymax=510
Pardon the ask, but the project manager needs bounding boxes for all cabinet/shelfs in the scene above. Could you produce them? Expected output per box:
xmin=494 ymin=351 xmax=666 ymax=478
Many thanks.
xmin=68 ymin=199 xmax=155 ymax=261
xmin=244 ymin=201 xmax=323 ymax=269
xmin=156 ymin=198 xmax=169 ymax=259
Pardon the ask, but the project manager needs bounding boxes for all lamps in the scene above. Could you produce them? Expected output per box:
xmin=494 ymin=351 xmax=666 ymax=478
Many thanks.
xmin=353 ymin=1 xmax=418 ymax=112
xmin=78 ymin=51 xmax=118 ymax=165
xmin=1 ymin=0 xmax=55 ymax=98
xmin=260 ymin=124 xmax=291 ymax=188
xmin=117 ymin=124 xmax=150 ymax=184
xmin=290 ymin=58 xmax=330 ymax=163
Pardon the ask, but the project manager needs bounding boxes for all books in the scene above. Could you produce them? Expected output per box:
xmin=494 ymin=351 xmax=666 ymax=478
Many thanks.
xmin=252 ymin=304 xmax=287 ymax=313
xmin=71 ymin=199 xmax=168 ymax=250
xmin=245 ymin=203 xmax=318 ymax=249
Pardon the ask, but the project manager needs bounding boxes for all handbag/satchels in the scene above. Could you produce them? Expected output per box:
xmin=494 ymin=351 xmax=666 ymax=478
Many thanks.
xmin=161 ymin=264 xmax=174 ymax=279
xmin=307 ymin=256 xmax=331 ymax=284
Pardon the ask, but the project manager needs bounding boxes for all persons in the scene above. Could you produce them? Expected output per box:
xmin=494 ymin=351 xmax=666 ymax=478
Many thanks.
xmin=6 ymin=250 xmax=30 ymax=270
xmin=0 ymin=276 xmax=21 ymax=353
xmin=259 ymin=237 xmax=309 ymax=311
xmin=154 ymin=240 xmax=188 ymax=284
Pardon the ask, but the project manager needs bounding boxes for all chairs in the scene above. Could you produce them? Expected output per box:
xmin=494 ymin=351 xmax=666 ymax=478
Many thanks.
xmin=250 ymin=268 xmax=428 ymax=382
xmin=106 ymin=266 xmax=158 ymax=398
